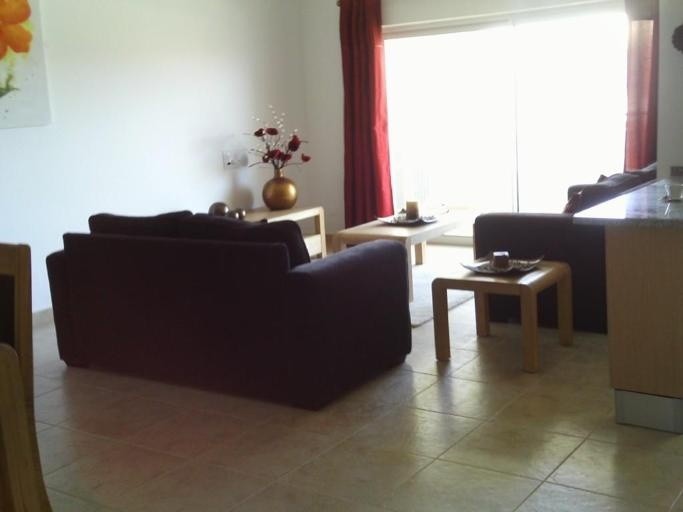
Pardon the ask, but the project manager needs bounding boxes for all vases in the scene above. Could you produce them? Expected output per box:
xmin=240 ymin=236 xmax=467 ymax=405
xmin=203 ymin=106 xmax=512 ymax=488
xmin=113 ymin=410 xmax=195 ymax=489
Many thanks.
xmin=260 ymin=168 xmax=298 ymax=207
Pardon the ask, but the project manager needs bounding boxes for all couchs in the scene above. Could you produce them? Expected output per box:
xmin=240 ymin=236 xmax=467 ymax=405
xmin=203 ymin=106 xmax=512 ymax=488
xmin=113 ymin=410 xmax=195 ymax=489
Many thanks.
xmin=472 ymin=162 xmax=660 ymax=334
xmin=44 ymin=210 xmax=412 ymax=410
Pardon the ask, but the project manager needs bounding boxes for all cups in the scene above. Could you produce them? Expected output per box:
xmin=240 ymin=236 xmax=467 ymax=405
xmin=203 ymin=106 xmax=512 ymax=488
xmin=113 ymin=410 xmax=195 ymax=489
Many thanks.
xmin=491 ymin=250 xmax=509 ymax=269
xmin=404 ymin=200 xmax=419 ymax=220
xmin=664 ymin=184 xmax=683 ymax=202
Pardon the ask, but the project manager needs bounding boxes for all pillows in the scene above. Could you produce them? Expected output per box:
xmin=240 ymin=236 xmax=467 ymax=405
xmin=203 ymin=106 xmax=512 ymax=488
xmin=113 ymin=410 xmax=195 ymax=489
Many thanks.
xmin=88 ymin=209 xmax=309 ymax=268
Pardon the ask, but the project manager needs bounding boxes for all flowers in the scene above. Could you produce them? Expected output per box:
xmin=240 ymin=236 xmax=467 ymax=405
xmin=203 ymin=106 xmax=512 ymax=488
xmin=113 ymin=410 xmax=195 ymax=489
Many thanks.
xmin=241 ymin=106 xmax=310 ymax=168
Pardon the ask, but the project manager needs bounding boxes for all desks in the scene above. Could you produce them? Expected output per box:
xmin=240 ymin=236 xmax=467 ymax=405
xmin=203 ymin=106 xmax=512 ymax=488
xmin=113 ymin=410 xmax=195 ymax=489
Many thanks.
xmin=432 ymin=259 xmax=572 ymax=373
xmin=235 ymin=205 xmax=327 ymax=260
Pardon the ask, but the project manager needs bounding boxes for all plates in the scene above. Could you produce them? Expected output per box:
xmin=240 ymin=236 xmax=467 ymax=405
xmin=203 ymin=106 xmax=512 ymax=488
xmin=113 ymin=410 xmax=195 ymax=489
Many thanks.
xmin=372 ymin=208 xmax=440 ymax=226
xmin=458 ymin=254 xmax=545 ymax=275
